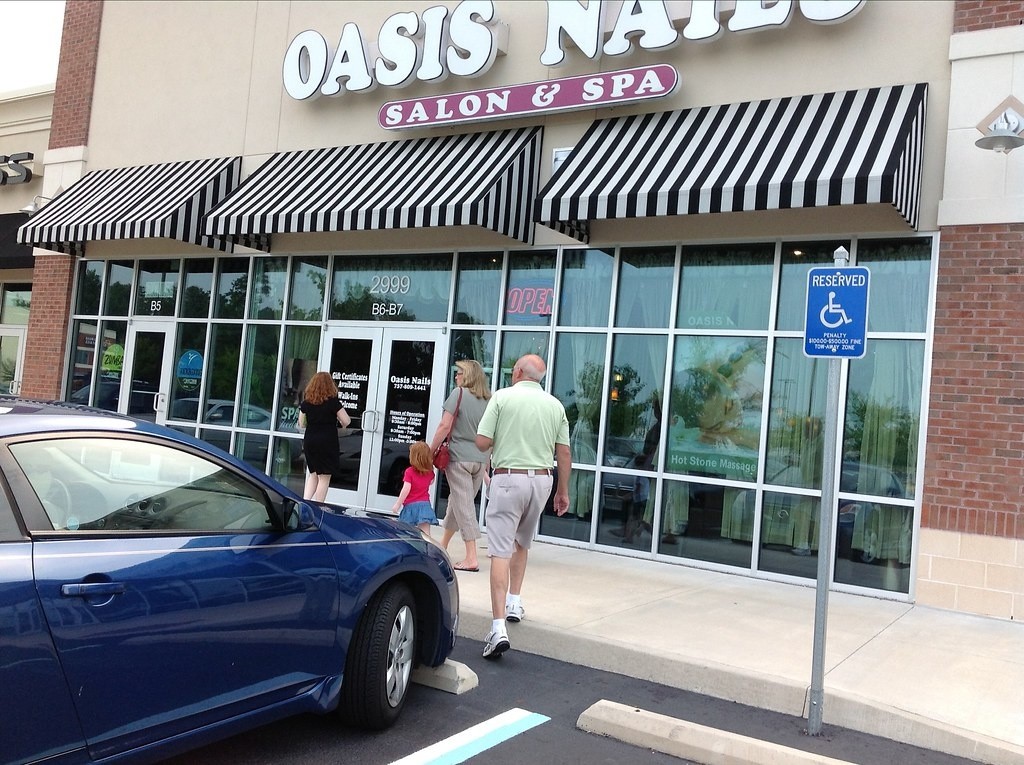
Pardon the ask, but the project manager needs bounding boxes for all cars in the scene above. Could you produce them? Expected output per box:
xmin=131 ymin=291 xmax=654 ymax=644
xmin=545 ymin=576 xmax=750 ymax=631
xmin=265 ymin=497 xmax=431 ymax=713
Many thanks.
xmin=70 ymin=382 xmax=408 ymax=494
xmin=600 ymin=437 xmax=910 ymax=565
xmin=0 ymin=389 xmax=459 ymax=765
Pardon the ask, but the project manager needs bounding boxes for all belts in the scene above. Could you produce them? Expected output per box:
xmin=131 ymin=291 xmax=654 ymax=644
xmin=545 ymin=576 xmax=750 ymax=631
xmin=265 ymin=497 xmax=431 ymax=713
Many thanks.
xmin=494 ymin=469 xmax=553 ymax=474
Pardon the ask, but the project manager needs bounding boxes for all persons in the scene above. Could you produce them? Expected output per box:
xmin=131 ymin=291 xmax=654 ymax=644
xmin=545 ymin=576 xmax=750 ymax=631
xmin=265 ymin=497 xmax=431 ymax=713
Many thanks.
xmin=608 ymin=398 xmax=686 ymax=545
xmin=430 ymin=360 xmax=493 ymax=572
xmin=475 ymin=354 xmax=571 ymax=659
xmin=669 ymin=337 xmax=768 ymax=450
xmin=393 ymin=442 xmax=439 ymax=537
xmin=298 ymin=372 xmax=351 ymax=503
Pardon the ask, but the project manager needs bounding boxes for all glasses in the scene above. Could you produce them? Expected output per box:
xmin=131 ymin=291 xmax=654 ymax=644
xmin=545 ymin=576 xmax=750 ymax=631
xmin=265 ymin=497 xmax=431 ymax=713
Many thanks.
xmin=456 ymin=372 xmax=463 ymax=376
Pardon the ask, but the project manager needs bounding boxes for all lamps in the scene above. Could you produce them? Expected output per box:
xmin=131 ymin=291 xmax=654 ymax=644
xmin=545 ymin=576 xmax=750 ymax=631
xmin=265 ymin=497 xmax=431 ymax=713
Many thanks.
xmin=976 ymin=112 xmax=1024 ymax=154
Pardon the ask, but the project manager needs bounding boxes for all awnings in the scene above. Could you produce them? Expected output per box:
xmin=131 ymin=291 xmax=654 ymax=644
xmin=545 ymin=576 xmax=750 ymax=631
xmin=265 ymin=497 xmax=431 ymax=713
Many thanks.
xmin=532 ymin=83 xmax=928 ymax=244
xmin=17 ymin=155 xmax=243 ymax=258
xmin=199 ymin=125 xmax=544 ymax=253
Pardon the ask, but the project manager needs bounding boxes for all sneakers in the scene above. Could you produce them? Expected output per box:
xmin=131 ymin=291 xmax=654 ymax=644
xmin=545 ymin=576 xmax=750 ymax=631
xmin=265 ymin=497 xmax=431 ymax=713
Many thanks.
xmin=482 ymin=630 xmax=511 ymax=658
xmin=505 ymin=603 xmax=525 ymax=622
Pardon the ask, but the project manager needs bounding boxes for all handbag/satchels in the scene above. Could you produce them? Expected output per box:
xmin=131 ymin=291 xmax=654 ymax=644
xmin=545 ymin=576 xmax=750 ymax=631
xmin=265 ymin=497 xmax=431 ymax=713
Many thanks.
xmin=432 ymin=442 xmax=450 ymax=471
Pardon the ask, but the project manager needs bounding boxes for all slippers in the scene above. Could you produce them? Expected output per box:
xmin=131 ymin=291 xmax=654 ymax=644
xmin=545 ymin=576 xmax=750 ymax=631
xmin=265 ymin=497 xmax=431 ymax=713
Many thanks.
xmin=453 ymin=560 xmax=480 ymax=572
